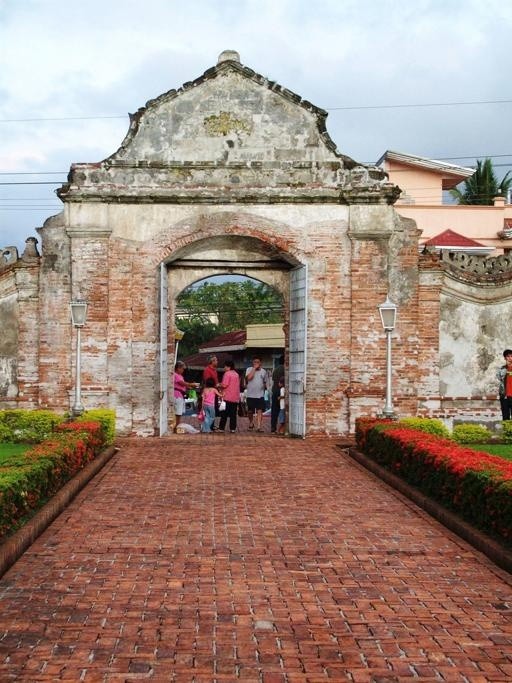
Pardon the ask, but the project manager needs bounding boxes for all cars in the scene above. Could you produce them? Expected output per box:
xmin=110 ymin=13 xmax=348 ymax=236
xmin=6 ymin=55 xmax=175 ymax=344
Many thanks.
xmin=184 ymin=394 xmax=227 ymax=414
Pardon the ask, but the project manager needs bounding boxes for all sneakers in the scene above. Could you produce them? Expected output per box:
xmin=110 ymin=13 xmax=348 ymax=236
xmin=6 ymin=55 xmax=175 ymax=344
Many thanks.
xmin=271 ymin=431 xmax=288 ymax=436
xmin=215 ymin=429 xmax=224 ymax=433
xmin=230 ymin=430 xmax=235 ymax=433
xmin=248 ymin=424 xmax=254 ymax=432
xmin=256 ymin=428 xmax=264 ymax=433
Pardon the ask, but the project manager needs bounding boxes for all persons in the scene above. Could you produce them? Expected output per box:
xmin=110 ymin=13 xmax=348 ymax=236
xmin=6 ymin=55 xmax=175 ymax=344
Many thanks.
xmin=174 ymin=353 xmax=285 ymax=433
xmin=496 ymin=349 xmax=512 ymax=421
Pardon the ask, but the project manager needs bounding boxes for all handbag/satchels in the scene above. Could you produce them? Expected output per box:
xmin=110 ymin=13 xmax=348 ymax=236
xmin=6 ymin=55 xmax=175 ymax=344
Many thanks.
xmin=184 ymin=393 xmax=189 ymax=399
xmin=198 ymin=409 xmax=204 ymax=421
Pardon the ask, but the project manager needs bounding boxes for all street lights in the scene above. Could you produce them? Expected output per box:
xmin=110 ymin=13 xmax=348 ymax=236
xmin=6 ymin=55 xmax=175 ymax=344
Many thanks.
xmin=69 ymin=290 xmax=88 ymax=416
xmin=376 ymin=293 xmax=400 ymax=421
